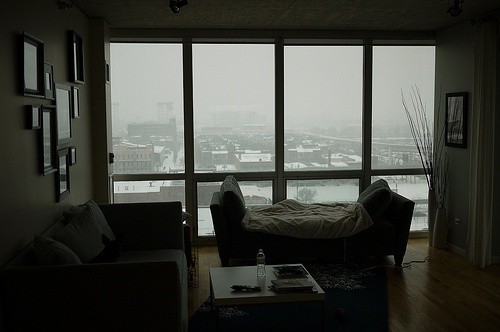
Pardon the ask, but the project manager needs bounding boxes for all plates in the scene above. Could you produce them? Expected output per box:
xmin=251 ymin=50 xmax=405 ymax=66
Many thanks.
xmin=271 ymin=265 xmax=309 ymax=279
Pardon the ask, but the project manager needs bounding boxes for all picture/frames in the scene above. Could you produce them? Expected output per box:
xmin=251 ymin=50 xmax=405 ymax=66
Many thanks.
xmin=17 ymin=29 xmax=85 ymax=203
xmin=444 ymin=92 xmax=469 ymax=149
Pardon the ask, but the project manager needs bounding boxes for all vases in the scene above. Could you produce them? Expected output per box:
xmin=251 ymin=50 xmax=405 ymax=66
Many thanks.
xmin=428 ymin=189 xmax=449 ymax=250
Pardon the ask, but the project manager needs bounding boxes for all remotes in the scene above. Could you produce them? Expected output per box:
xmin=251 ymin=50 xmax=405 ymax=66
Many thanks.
xmin=231 ymin=285 xmax=261 ymax=292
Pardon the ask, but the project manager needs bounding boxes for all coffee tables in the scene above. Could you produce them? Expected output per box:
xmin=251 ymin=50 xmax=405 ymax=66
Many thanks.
xmin=209 ymin=263 xmax=325 ymax=332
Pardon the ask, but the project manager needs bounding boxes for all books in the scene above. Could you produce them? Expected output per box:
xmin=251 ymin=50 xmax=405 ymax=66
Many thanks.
xmin=271 ymin=278 xmax=318 ymax=294
xmin=271 ymin=266 xmax=309 ymax=279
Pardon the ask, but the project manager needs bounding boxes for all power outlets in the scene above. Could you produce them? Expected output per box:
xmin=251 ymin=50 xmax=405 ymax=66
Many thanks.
xmin=456 ymin=219 xmax=459 ymax=225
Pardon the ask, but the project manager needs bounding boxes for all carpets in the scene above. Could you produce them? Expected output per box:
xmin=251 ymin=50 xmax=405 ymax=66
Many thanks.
xmin=184 ymin=259 xmax=388 ymax=332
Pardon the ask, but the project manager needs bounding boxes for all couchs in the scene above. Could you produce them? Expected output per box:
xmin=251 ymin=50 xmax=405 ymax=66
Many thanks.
xmin=0 ymin=202 xmax=190 ymax=332
xmin=208 ymin=175 xmax=415 ymax=266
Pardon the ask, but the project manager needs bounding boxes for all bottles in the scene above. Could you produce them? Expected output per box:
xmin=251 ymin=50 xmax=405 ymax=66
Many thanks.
xmin=257 ymin=249 xmax=266 ymax=277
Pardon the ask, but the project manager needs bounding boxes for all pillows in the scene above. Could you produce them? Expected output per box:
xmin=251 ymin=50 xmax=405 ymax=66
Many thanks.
xmin=22 ymin=199 xmax=116 ymax=266
